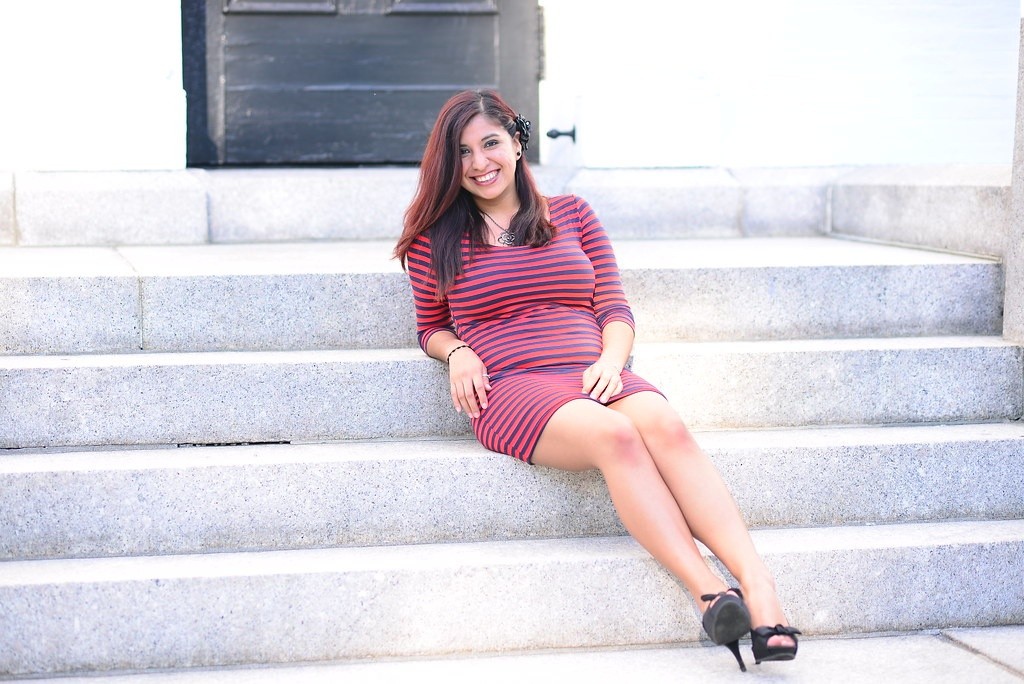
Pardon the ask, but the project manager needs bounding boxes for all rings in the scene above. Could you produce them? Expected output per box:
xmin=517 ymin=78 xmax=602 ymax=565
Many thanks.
xmin=482 ymin=373 xmax=491 ymax=379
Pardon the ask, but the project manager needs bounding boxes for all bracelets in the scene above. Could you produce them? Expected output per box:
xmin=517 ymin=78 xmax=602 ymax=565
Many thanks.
xmin=447 ymin=345 xmax=475 ymax=363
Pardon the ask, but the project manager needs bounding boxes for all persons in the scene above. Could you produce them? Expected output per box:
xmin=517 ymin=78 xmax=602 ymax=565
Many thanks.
xmin=389 ymin=88 xmax=803 ymax=672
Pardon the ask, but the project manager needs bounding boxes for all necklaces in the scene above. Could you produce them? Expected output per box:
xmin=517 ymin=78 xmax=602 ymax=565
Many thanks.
xmin=480 ymin=207 xmax=516 ymax=245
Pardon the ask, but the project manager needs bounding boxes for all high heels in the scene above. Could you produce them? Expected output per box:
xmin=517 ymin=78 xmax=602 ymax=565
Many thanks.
xmin=749 ymin=624 xmax=798 ymax=665
xmin=702 ymin=594 xmax=751 ymax=673
